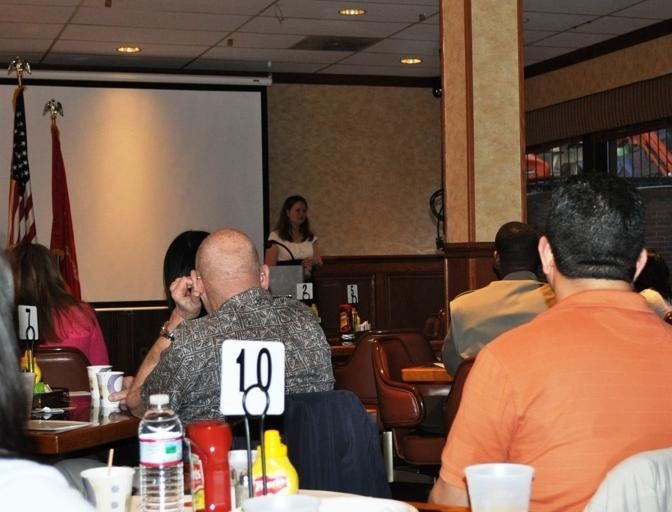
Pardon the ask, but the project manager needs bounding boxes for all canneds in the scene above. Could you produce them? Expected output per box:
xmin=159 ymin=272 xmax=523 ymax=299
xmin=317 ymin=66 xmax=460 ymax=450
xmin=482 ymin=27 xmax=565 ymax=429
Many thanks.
xmin=338 ymin=304 xmax=352 ymax=334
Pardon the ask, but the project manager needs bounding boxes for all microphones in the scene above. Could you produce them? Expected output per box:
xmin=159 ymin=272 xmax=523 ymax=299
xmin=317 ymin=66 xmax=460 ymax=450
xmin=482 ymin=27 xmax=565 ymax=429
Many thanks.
xmin=266 ymin=239 xmax=294 ymax=260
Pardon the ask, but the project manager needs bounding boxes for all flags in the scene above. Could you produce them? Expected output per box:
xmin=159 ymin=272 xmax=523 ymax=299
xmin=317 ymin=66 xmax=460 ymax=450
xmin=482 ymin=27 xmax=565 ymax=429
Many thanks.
xmin=8 ymin=86 xmax=39 ymax=248
xmin=48 ymin=124 xmax=82 ymax=301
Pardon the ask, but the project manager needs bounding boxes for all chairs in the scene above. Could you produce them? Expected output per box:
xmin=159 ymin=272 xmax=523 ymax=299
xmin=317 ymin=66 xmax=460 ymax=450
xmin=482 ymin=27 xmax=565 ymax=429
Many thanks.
xmin=443 ymin=356 xmax=475 ymax=434
xmin=371 ymin=334 xmax=452 ymax=486
xmin=422 ymin=312 xmax=446 ymax=336
xmin=33 ymin=343 xmax=89 ymax=390
xmin=333 ymin=326 xmax=438 ymax=405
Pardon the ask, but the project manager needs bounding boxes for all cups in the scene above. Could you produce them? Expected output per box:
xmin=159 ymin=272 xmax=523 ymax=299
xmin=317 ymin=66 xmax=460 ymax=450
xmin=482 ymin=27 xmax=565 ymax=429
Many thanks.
xmin=462 ymin=463 xmax=534 ymax=511
xmin=86 ymin=364 xmax=114 ymax=400
xmin=226 ymin=449 xmax=257 ymax=504
xmin=20 ymin=373 xmax=38 ymax=417
xmin=80 ymin=467 xmax=135 ymax=510
xmin=96 ymin=372 xmax=124 ymax=408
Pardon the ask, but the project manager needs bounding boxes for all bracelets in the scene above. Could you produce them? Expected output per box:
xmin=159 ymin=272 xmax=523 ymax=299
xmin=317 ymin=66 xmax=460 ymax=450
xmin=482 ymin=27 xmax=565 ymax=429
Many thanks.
xmin=159 ymin=325 xmax=175 ymax=343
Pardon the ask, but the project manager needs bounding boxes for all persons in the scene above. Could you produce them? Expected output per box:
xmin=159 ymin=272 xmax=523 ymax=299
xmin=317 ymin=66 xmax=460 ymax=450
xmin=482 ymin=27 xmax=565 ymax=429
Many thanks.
xmin=443 ymin=222 xmax=556 ymax=376
xmin=263 ymin=195 xmax=325 ymax=333
xmin=639 ymin=257 xmax=672 ymax=326
xmin=108 ymin=228 xmax=335 ymax=452
xmin=582 ymin=446 xmax=672 ymax=512
xmin=8 ymin=243 xmax=109 ymax=368
xmin=158 ymin=230 xmax=211 ymax=323
xmin=1 ymin=290 xmax=99 ymax=512
xmin=425 ymin=171 xmax=672 ymax=512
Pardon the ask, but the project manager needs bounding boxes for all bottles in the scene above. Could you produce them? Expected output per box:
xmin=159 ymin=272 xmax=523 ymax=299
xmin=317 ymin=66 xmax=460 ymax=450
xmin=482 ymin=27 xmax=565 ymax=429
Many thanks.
xmin=311 ymin=301 xmax=372 ymax=336
xmin=22 ymin=349 xmax=41 ymax=384
xmin=251 ymin=428 xmax=298 ymax=498
xmin=139 ymin=395 xmax=184 ymax=511
xmin=187 ymin=421 xmax=232 ymax=511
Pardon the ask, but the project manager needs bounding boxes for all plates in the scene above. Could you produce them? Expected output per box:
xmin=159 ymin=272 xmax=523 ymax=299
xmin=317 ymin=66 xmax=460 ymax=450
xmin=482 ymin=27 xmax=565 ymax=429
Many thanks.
xmin=36 ymin=386 xmax=70 ymax=405
